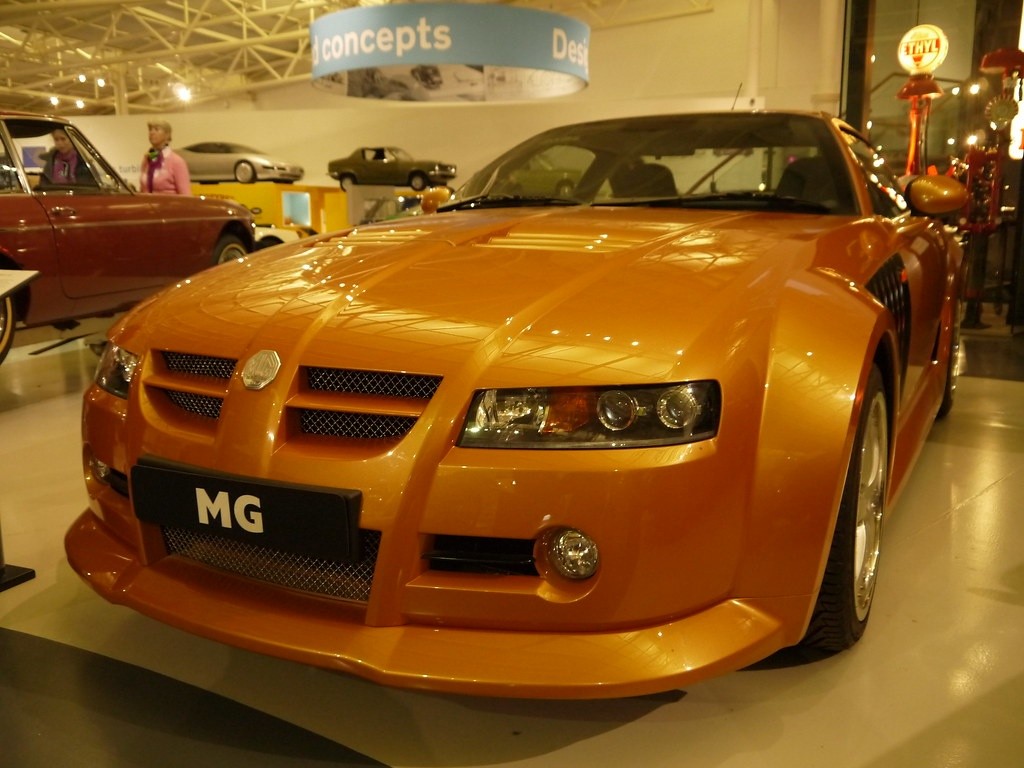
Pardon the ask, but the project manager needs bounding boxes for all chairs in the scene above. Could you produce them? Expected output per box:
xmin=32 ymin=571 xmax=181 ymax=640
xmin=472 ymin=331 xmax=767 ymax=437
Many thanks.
xmin=609 ymin=164 xmax=680 ymax=196
xmin=773 ymin=155 xmax=849 ymax=205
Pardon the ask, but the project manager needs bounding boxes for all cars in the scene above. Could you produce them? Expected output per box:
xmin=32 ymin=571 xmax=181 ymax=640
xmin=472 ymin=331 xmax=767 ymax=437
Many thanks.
xmin=328 ymin=146 xmax=457 ymax=192
xmin=483 ymin=151 xmax=581 ymax=199
xmin=176 ymin=142 xmax=304 ymax=185
xmin=0 ymin=109 xmax=255 ymax=366
xmin=64 ymin=110 xmax=968 ymax=698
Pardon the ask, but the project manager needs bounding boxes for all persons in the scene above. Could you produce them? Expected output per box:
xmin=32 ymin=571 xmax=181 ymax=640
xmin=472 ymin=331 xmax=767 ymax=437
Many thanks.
xmin=38 ymin=129 xmax=97 ymax=186
xmin=140 ymin=121 xmax=191 ymax=196
